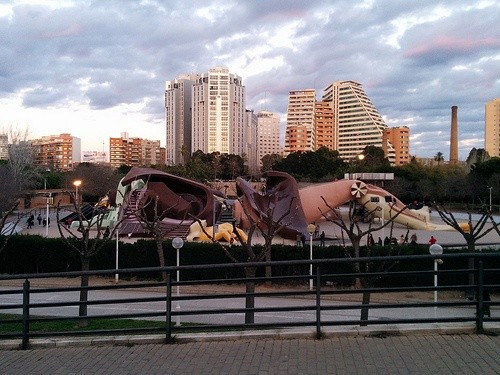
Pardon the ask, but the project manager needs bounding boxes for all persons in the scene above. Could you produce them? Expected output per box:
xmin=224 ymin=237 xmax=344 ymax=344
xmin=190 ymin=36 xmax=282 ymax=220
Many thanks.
xmin=297 ymin=224 xmax=326 ymax=247
xmin=26 ymin=213 xmax=71 ymax=229
xmin=368 ymin=231 xmax=436 ymax=245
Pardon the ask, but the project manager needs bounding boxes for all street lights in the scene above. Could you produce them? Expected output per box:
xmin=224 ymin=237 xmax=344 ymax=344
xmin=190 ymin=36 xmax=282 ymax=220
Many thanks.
xmin=429 ymin=244 xmax=443 ymax=319
xmin=172 ymin=237 xmax=184 ymax=326
xmin=306 ymin=223 xmax=316 ymax=290
xmin=113 ymin=220 xmax=122 ymax=285
xmin=72 ymin=180 xmax=81 ymax=206
